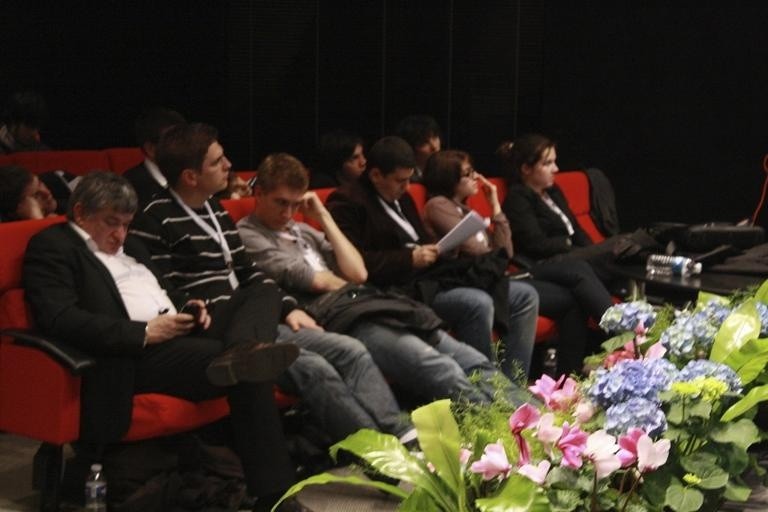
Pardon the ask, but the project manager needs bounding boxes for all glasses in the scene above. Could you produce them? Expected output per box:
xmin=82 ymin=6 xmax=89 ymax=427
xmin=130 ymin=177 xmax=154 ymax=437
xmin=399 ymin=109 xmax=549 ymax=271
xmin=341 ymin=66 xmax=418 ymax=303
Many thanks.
xmin=460 ymin=166 xmax=473 ymax=177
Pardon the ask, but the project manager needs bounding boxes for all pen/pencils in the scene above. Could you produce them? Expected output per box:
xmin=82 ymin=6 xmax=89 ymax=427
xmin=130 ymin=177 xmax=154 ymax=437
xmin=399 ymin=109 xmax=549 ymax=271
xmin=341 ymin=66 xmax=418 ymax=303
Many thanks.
xmin=405 ymin=243 xmax=421 ymax=249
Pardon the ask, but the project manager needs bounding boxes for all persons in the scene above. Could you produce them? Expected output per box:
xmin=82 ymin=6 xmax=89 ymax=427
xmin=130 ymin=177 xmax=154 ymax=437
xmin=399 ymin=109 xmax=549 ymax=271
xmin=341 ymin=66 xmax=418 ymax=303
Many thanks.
xmin=123 ymin=120 xmax=419 ymax=452
xmin=123 ymin=107 xmax=253 ymax=208
xmin=397 ymin=111 xmax=442 ymax=184
xmin=235 ymin=151 xmax=548 ymax=419
xmin=247 ymin=141 xmax=311 ymax=188
xmin=0 ymin=165 xmax=89 ymax=221
xmin=502 ymin=133 xmax=616 ymax=371
xmin=324 ymin=134 xmax=540 ymax=390
xmin=419 ymin=151 xmax=540 ymax=383
xmin=21 ymin=170 xmax=301 ymax=512
xmin=324 ymin=137 xmax=369 ymax=187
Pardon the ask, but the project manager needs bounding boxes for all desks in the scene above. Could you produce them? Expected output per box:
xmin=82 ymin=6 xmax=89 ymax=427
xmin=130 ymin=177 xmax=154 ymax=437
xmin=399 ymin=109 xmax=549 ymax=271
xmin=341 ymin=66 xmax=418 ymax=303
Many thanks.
xmin=689 ymin=222 xmax=766 ymax=251
xmin=604 ymin=248 xmax=765 ymax=298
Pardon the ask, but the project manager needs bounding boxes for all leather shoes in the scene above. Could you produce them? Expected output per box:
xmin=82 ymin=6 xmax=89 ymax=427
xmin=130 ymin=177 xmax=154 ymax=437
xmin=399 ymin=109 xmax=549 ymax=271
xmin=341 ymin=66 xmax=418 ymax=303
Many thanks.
xmin=207 ymin=342 xmax=299 ymax=387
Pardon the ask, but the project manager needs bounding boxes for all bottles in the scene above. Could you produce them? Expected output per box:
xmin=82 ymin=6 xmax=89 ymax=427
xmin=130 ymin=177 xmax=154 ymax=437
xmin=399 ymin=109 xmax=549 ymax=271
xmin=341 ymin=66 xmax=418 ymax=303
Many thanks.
xmin=542 ymin=348 xmax=558 ymax=377
xmin=646 ymin=255 xmax=704 ymax=278
xmin=86 ymin=464 xmax=107 ymax=512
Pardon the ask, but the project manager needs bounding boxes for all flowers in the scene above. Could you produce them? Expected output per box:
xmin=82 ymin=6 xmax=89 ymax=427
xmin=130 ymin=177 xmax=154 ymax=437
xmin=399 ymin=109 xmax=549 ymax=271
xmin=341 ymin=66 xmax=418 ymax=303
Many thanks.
xmin=670 ymin=276 xmax=768 ymax=510
